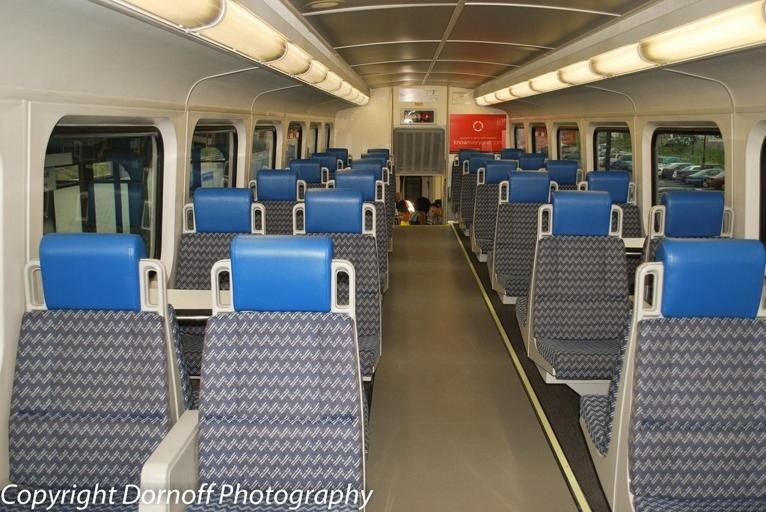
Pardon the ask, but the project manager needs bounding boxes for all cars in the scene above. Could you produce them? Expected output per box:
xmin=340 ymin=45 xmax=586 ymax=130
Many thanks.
xmin=539 ymin=145 xmax=725 ymax=189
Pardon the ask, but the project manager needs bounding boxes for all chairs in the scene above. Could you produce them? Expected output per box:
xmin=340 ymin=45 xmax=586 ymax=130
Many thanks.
xmin=2 ymin=144 xmax=394 ymax=511
xmin=443 ymin=145 xmax=764 ymax=512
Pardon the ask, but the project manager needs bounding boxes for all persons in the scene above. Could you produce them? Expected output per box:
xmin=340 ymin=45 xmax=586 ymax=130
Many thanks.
xmin=396 ymin=195 xmax=444 ymax=225
